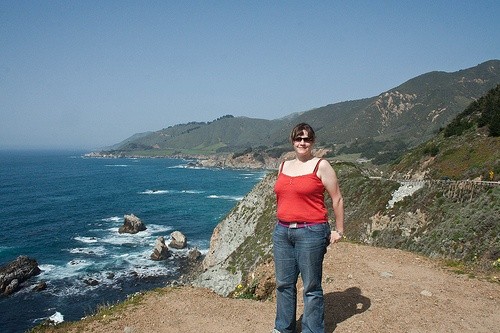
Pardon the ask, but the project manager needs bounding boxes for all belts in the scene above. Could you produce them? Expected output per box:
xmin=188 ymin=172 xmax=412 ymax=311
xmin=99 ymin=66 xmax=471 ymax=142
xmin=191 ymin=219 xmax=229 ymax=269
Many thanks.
xmin=278 ymin=219 xmax=326 ymax=228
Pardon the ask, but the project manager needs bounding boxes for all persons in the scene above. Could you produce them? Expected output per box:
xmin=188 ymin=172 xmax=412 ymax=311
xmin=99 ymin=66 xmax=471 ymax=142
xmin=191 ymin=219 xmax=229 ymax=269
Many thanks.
xmin=273 ymin=123 xmax=344 ymax=333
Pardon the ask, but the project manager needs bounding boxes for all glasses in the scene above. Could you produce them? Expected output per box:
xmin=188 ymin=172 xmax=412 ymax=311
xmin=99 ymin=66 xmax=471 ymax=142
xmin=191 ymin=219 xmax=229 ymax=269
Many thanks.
xmin=293 ymin=136 xmax=311 ymax=142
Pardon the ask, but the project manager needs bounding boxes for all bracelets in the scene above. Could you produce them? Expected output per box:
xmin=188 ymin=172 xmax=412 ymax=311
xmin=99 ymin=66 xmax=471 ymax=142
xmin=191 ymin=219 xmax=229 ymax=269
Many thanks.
xmin=336 ymin=230 xmax=344 ymax=237
